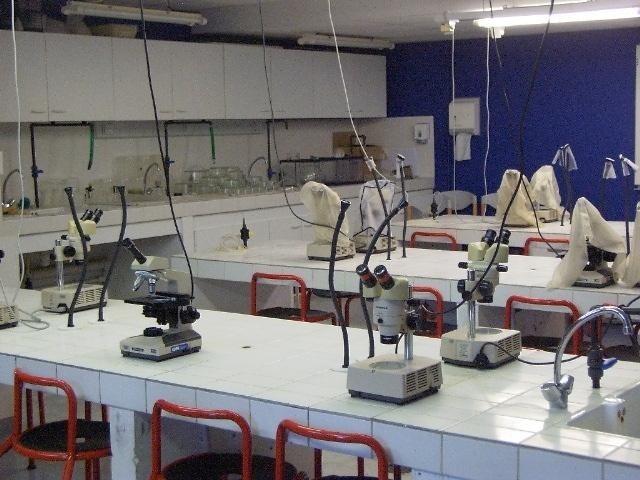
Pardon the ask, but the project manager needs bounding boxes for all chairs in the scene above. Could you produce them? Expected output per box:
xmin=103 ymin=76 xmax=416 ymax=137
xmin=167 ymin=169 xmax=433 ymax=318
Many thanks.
xmin=274 ymin=419 xmax=389 ymax=480
xmin=2 ymin=365 xmax=113 ymax=479
xmin=146 ymin=397 xmax=295 ymax=479
xmin=438 ymin=187 xmax=498 ymax=216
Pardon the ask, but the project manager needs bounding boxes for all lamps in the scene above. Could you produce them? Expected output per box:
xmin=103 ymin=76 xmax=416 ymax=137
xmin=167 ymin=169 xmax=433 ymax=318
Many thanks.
xmin=296 ymin=35 xmax=396 ymax=50
xmin=63 ymin=3 xmax=207 ymax=26
xmin=471 ymin=0 xmax=640 ymax=30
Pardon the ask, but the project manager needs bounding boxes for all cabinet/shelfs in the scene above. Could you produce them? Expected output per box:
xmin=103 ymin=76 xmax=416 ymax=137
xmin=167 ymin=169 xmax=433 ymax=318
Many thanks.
xmin=313 ymin=49 xmax=387 ymax=118
xmin=225 ymin=42 xmax=314 ymax=119
xmin=112 ymin=37 xmax=225 ymax=119
xmin=2 ymin=28 xmax=116 ymax=121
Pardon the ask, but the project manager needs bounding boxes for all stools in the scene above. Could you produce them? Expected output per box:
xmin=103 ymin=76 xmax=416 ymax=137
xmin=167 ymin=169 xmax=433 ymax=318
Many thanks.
xmin=250 ymin=273 xmax=332 ymax=325
xmin=408 ymin=288 xmax=444 ymax=339
xmin=523 ymin=239 xmax=569 ymax=258
xmin=504 ymin=294 xmax=578 ymax=355
xmin=410 ymin=232 xmax=458 ymax=250
xmin=302 ymin=288 xmax=362 ymax=327
xmin=597 ymin=302 xmax=639 ymax=352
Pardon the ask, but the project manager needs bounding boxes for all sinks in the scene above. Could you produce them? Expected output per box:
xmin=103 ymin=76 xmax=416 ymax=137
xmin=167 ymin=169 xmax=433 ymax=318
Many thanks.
xmin=566 ymin=386 xmax=638 ymax=437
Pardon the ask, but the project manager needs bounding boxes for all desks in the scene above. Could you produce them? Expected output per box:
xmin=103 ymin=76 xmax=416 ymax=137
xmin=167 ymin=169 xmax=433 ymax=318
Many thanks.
xmin=392 ymin=213 xmax=639 ymax=250
xmin=2 ymin=299 xmax=640 ymax=479
xmin=170 ymin=238 xmax=640 ymax=351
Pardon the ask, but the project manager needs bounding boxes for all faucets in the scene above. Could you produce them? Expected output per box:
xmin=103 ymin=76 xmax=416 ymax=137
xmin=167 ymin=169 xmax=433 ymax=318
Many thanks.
xmin=2 ymin=169 xmax=20 ymax=202
xmin=248 ymin=157 xmax=267 ymax=178
xmin=542 ymin=305 xmax=635 ymax=406
xmin=143 ymin=162 xmax=160 ymax=193
